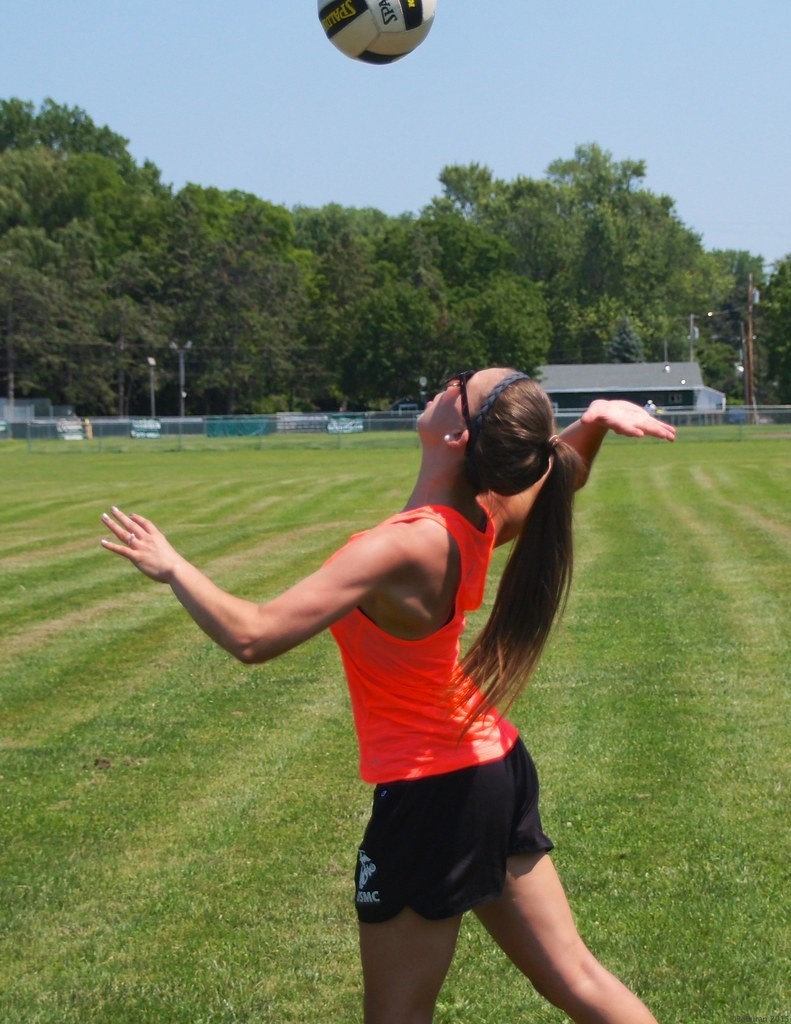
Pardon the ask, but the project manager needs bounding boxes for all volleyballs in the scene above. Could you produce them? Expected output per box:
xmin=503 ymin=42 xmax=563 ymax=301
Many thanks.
xmin=318 ymin=0 xmax=437 ymax=65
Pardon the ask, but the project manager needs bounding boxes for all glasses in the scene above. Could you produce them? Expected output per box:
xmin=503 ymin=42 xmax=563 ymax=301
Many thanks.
xmin=444 ymin=370 xmax=475 ymax=436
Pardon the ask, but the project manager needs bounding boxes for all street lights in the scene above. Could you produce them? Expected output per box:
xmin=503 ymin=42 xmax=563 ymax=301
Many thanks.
xmin=170 ymin=339 xmax=192 ymax=417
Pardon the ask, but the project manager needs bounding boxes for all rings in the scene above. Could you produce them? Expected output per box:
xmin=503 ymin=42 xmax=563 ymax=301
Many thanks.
xmin=128 ymin=534 xmax=136 ymax=543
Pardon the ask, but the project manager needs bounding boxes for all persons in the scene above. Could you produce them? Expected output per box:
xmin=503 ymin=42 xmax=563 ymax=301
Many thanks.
xmin=645 ymin=400 xmax=656 ymax=413
xmin=95 ymin=369 xmax=677 ymax=1022
